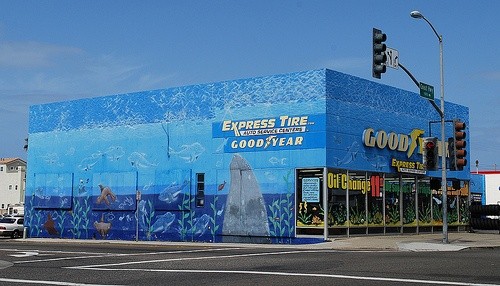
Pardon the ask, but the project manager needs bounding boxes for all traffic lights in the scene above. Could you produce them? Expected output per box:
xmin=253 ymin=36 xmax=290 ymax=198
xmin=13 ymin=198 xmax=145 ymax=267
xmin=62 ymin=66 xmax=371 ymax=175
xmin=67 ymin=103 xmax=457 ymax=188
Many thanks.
xmin=372 ymin=28 xmax=387 ymax=79
xmin=423 ymin=136 xmax=439 ymax=171
xmin=453 ymin=118 xmax=467 ymax=171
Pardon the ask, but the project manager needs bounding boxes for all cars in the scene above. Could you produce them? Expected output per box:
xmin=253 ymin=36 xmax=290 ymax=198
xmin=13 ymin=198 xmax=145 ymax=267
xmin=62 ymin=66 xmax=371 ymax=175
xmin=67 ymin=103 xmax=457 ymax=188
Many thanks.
xmin=0 ymin=216 xmax=24 ymax=239
